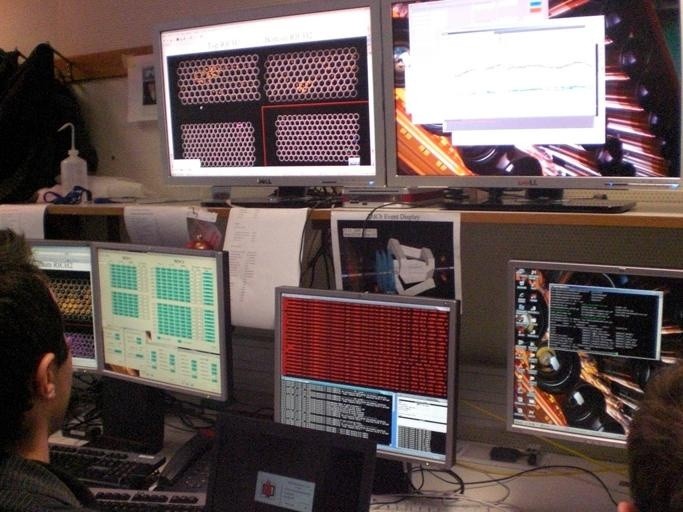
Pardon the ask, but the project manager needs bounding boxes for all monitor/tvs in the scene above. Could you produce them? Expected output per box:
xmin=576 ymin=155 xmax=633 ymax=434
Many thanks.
xmin=152 ymin=0 xmax=386 ymax=208
xmin=24 ymin=238 xmax=99 ymax=373
xmin=91 ymin=242 xmax=229 ymax=403
xmin=506 ymin=259 xmax=683 ymax=449
xmin=379 ymin=0 xmax=683 ymax=213
xmin=274 ymin=285 xmax=460 ymax=494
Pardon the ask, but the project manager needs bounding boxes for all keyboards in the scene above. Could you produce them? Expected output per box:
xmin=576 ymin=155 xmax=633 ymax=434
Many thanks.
xmin=48 ymin=442 xmax=167 ymax=491
xmin=89 ymin=487 xmax=207 ymax=512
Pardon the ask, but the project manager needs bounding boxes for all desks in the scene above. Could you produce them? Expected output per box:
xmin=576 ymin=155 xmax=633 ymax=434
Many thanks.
xmin=40 ymin=384 xmax=638 ymax=511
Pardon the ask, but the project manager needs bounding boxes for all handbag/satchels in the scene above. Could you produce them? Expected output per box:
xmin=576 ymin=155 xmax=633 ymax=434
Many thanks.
xmin=0 ymin=44 xmax=97 ymax=203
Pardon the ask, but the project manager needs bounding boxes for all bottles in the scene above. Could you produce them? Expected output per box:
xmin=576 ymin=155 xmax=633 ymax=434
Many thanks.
xmin=59 ymin=148 xmax=87 ymax=202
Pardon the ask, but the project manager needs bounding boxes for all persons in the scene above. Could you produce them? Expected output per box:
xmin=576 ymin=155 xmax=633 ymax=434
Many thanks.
xmin=0 ymin=228 xmax=93 ymax=510
xmin=616 ymin=359 xmax=682 ymax=511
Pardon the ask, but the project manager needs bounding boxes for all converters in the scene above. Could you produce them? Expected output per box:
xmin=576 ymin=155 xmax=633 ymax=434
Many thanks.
xmin=491 ymin=447 xmax=517 ymax=461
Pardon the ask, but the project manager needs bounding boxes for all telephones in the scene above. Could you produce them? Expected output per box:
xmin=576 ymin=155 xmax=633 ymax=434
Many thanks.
xmin=156 ymin=428 xmax=220 ymax=490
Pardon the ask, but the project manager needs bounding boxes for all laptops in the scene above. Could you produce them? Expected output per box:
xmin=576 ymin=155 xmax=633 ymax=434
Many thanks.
xmin=204 ymin=413 xmax=377 ymax=512
